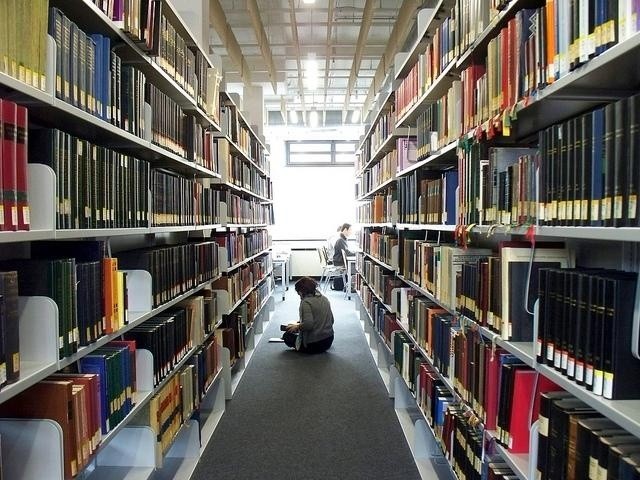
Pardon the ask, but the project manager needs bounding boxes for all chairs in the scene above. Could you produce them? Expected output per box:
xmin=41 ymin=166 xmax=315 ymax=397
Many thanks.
xmin=316 ymin=240 xmax=347 ymax=294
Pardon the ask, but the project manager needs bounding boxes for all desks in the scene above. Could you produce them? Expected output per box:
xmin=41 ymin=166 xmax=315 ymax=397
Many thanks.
xmin=272 ymin=251 xmax=293 ymax=302
xmin=346 ymin=256 xmax=356 ymax=300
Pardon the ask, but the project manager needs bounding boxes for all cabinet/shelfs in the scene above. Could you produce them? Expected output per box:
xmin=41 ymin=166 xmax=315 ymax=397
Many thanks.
xmin=354 ymin=0 xmax=634 ymax=480
xmin=1 ymin=1 xmax=277 ymax=479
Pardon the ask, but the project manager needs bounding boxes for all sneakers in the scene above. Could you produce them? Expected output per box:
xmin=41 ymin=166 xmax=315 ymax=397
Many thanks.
xmin=344 ymin=287 xmax=357 ymax=293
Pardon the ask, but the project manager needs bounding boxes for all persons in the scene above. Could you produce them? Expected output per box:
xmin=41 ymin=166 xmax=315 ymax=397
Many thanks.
xmin=326 ymin=221 xmax=355 ymax=271
xmin=280 ymin=277 xmax=335 ymax=355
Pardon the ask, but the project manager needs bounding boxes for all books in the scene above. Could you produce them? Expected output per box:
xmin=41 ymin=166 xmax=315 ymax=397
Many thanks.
xmin=356 ymin=1 xmax=639 ymax=225
xmin=358 ymin=227 xmax=638 ymax=479
xmin=2 ymin=2 xmax=272 ymax=230
xmin=280 ymin=324 xmax=300 ymax=333
xmin=1 ymin=233 xmax=275 ymax=480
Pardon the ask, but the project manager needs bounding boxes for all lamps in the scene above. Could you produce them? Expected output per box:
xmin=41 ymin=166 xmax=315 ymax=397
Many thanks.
xmin=310 ymin=91 xmax=317 ymax=129
